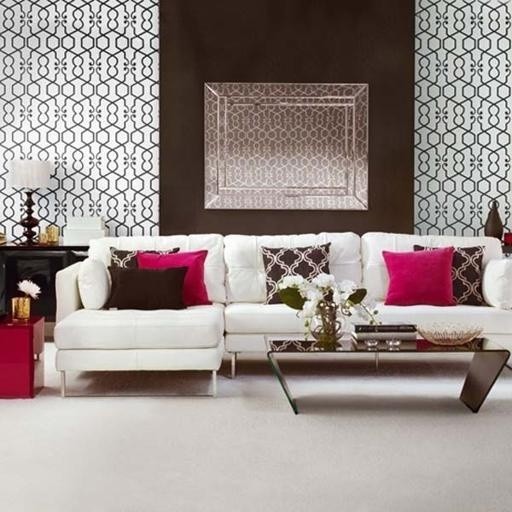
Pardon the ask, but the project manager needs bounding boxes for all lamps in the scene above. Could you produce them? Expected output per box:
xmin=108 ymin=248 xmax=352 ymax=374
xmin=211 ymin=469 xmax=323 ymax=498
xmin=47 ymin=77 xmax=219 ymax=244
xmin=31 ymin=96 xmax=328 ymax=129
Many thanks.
xmin=10 ymin=160 xmax=50 ymax=248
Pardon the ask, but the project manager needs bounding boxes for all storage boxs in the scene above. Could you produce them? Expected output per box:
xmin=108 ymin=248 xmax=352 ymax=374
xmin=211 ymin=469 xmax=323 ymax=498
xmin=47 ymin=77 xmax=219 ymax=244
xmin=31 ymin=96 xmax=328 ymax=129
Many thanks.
xmin=68 ymin=217 xmax=104 ymax=230
xmin=63 ymin=228 xmax=108 ymax=245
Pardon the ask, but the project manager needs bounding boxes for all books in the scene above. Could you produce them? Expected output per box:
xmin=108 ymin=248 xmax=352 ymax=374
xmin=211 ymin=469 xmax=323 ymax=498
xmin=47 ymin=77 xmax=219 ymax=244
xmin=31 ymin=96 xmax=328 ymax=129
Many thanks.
xmin=350 ymin=322 xmax=418 ymax=341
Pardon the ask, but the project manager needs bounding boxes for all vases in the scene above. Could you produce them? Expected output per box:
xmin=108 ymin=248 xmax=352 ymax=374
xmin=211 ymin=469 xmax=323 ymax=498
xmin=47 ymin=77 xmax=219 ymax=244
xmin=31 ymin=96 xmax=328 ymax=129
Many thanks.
xmin=483 ymin=201 xmax=503 ymax=240
xmin=311 ymin=311 xmax=354 ymax=344
xmin=13 ymin=298 xmax=31 ymax=319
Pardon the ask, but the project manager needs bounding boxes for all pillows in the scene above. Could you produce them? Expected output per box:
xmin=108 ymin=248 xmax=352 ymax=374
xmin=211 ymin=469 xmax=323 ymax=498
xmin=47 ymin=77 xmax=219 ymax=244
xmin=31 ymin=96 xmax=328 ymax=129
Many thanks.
xmin=413 ymin=244 xmax=489 ymax=306
xmin=135 ymin=250 xmax=214 ymax=306
xmin=107 ymin=265 xmax=187 ymax=311
xmin=382 ymin=246 xmax=455 ymax=307
xmin=108 ymin=245 xmax=196 ymax=268
xmin=259 ymin=243 xmax=335 ymax=304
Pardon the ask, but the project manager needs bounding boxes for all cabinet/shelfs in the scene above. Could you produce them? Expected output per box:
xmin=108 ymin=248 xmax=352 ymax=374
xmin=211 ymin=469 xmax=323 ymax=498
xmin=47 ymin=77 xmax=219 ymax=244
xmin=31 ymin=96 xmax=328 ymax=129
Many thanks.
xmin=0 ymin=246 xmax=90 ymax=322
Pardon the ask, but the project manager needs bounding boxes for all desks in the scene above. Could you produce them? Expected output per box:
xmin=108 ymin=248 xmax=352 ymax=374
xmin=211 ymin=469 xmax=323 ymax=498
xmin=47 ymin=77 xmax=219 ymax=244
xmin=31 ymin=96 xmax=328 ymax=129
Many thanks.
xmin=0 ymin=318 xmax=46 ymax=399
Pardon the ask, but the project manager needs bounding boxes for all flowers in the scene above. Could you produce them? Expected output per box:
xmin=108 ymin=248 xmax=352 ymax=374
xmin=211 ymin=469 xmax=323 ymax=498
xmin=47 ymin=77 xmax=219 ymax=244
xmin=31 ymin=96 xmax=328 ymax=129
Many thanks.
xmin=279 ymin=273 xmax=384 ymax=326
xmin=19 ymin=279 xmax=43 ymax=299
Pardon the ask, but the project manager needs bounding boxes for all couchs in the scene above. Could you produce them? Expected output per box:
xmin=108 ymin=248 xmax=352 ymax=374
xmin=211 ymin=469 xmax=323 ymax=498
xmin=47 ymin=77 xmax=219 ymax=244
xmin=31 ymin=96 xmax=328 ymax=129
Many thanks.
xmin=362 ymin=233 xmax=512 ymax=370
xmin=57 ymin=234 xmax=226 ymax=397
xmin=222 ymin=234 xmax=364 ymax=376
